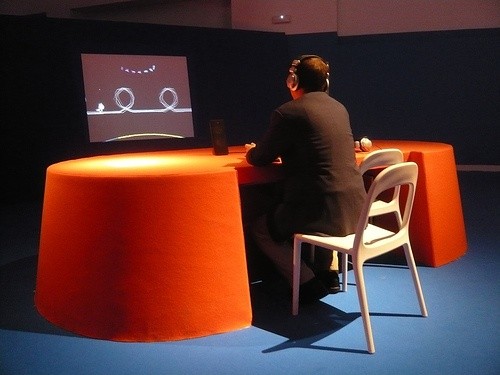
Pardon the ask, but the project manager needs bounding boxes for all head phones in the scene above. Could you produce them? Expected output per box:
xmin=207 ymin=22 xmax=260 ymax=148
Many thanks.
xmin=286 ymin=54 xmax=330 ymax=91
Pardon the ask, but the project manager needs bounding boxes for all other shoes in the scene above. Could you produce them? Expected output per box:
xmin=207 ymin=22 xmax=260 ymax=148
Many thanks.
xmin=323 ymin=271 xmax=340 ymax=294
xmin=278 ymin=277 xmax=330 ymax=308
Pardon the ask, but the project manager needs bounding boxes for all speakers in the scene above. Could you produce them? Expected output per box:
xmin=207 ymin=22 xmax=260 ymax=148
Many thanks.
xmin=209 ymin=118 xmax=228 ymax=155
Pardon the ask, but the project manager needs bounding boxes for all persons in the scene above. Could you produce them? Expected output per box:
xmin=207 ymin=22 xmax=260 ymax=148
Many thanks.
xmin=244 ymin=54 xmax=367 ymax=305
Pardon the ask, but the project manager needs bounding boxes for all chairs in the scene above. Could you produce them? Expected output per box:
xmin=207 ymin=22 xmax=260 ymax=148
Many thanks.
xmin=293 ymin=149 xmax=428 ymax=353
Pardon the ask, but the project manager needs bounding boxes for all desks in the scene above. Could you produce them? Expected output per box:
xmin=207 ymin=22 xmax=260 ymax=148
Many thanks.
xmin=36 ymin=139 xmax=467 ymax=344
